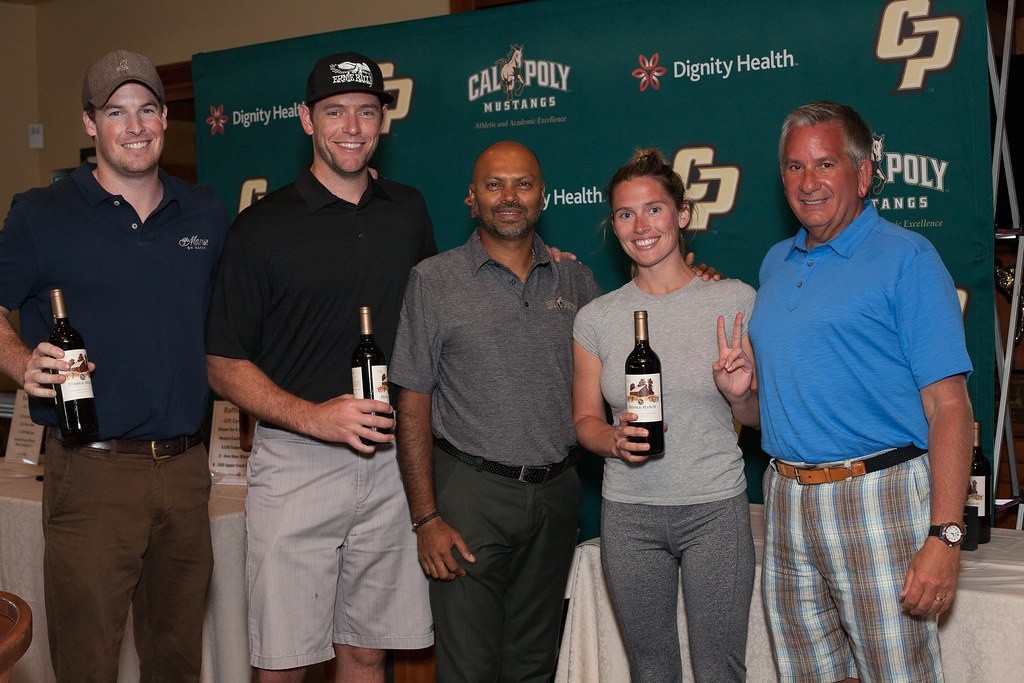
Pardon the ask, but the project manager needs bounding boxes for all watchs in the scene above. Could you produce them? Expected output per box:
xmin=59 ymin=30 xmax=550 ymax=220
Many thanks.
xmin=927 ymin=521 xmax=968 ymax=547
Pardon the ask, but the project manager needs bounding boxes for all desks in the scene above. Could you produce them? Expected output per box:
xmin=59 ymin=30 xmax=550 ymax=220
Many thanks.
xmin=554 ymin=504 xmax=1024 ymax=683
xmin=0 ymin=444 xmax=255 ymax=683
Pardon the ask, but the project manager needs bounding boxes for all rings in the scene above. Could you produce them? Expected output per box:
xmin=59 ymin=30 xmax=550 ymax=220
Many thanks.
xmin=935 ymin=596 xmax=945 ymax=602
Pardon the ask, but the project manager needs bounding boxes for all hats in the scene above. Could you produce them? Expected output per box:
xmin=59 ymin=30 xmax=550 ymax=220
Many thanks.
xmin=82 ymin=50 xmax=164 ymax=108
xmin=305 ymin=51 xmax=393 ymax=107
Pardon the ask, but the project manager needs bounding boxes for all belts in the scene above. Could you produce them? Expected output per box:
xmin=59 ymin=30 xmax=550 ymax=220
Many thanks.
xmin=434 ymin=436 xmax=583 ymax=484
xmin=49 ymin=427 xmax=202 ymax=460
xmin=776 ymin=445 xmax=929 ymax=485
xmin=257 ymin=416 xmax=281 ymax=430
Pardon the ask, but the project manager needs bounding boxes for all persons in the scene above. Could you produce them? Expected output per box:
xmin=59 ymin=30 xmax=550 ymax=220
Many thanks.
xmin=387 ymin=140 xmax=721 ymax=683
xmin=733 ymin=100 xmax=977 ymax=683
xmin=0 ymin=49 xmax=377 ymax=683
xmin=573 ymin=150 xmax=758 ymax=683
xmin=207 ymin=55 xmax=576 ymax=683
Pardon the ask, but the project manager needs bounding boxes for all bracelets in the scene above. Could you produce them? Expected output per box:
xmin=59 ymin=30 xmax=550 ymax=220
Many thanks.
xmin=412 ymin=510 xmax=440 ymax=535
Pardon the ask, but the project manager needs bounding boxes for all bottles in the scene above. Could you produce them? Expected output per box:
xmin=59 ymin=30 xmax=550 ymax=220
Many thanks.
xmin=351 ymin=306 xmax=394 ymax=446
xmin=624 ymin=311 xmax=665 ymax=456
xmin=964 ymin=423 xmax=990 ymax=544
xmin=47 ymin=289 xmax=98 ymax=445
